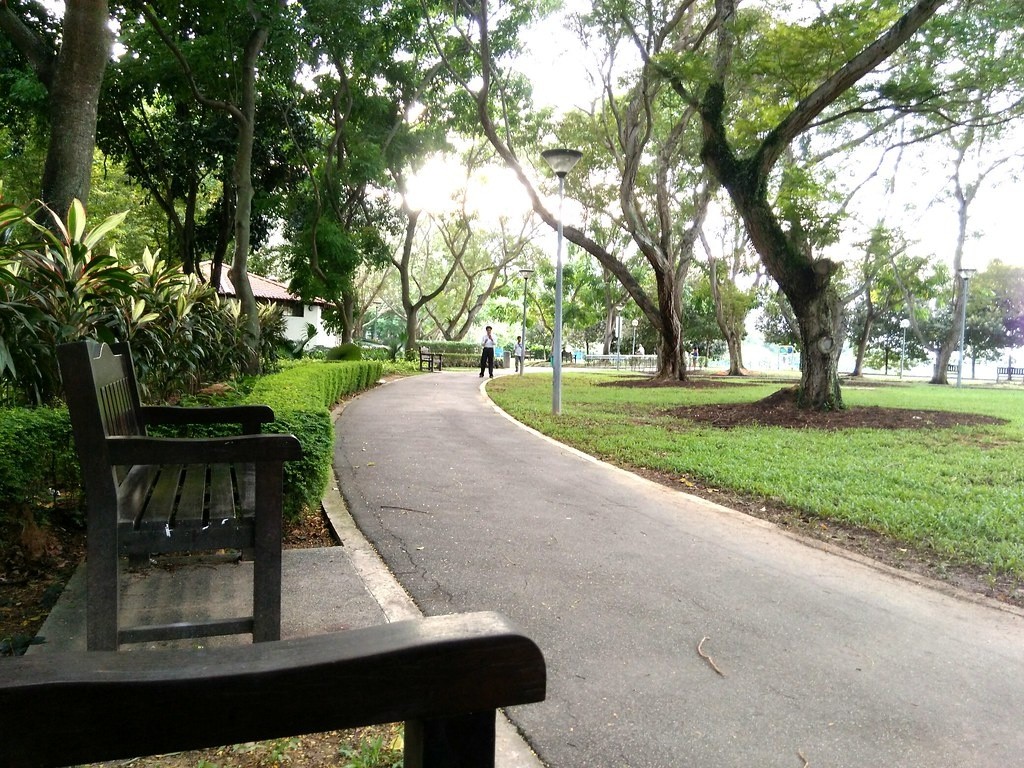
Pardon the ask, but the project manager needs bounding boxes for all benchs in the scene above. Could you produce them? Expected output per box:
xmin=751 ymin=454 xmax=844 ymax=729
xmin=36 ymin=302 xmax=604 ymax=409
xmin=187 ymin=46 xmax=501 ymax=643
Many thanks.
xmin=59 ymin=339 xmax=303 ymax=651
xmin=933 ymin=364 xmax=958 ymax=374
xmin=996 ymin=367 xmax=1024 ymax=382
xmin=419 ymin=345 xmax=442 ymax=371
xmin=1 ymin=612 xmax=546 ymax=768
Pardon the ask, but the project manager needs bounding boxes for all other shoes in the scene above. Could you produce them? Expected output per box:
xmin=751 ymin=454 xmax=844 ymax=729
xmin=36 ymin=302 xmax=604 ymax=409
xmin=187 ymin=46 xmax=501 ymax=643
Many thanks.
xmin=479 ymin=373 xmax=484 ymax=377
xmin=515 ymin=370 xmax=518 ymax=372
xmin=490 ymin=374 xmax=493 ymax=378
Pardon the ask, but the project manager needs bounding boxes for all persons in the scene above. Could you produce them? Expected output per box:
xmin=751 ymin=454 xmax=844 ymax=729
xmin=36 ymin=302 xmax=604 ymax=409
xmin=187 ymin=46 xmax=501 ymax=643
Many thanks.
xmin=691 ymin=347 xmax=697 ymax=367
xmin=479 ymin=326 xmax=497 ymax=378
xmin=636 ymin=344 xmax=645 ymax=354
xmin=512 ymin=335 xmax=522 ymax=372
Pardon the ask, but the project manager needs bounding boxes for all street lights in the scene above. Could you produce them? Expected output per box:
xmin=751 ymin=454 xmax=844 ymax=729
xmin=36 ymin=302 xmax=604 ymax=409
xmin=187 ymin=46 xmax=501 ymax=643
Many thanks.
xmin=956 ymin=268 xmax=977 ymax=389
xmin=899 ymin=318 xmax=910 ymax=379
xmin=631 ymin=319 xmax=638 ymax=355
xmin=614 ymin=305 xmax=625 ymax=370
xmin=518 ymin=268 xmax=535 ymax=376
xmin=539 ymin=147 xmax=583 ymax=418
